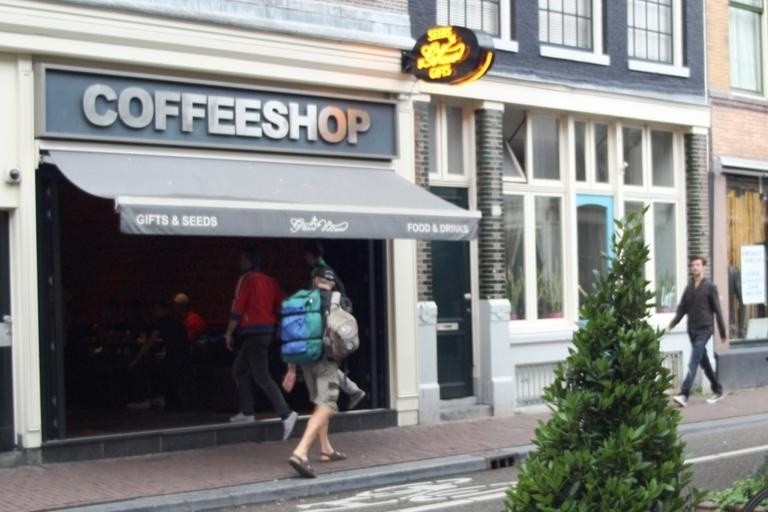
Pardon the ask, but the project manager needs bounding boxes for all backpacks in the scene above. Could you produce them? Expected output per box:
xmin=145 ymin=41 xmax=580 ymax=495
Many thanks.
xmin=280 ymin=287 xmax=322 ymax=363
xmin=326 ymin=291 xmax=361 ymax=361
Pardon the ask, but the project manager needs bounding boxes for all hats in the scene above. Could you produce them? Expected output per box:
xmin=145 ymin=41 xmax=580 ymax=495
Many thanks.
xmin=312 ymin=267 xmax=336 ymax=282
xmin=167 ymin=294 xmax=188 ymax=306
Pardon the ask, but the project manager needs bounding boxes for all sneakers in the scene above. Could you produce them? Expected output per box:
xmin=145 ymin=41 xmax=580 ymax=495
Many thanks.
xmin=675 ymin=394 xmax=687 ymax=406
xmin=707 ymin=393 xmax=723 ymax=403
xmin=283 ymin=411 xmax=298 ymax=441
xmin=347 ymin=390 xmax=367 ymax=410
xmin=127 ymin=400 xmax=152 ymax=410
xmin=230 ymin=412 xmax=255 ymax=422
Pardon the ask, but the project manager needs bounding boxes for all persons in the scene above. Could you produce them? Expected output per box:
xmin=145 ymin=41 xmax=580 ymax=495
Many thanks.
xmin=664 ymin=256 xmax=727 ymax=407
xmin=224 ymin=247 xmax=298 ymax=442
xmin=63 ymin=286 xmax=212 ymax=413
xmin=282 ymin=265 xmax=347 ymax=480
xmin=296 ymin=242 xmax=367 ymax=410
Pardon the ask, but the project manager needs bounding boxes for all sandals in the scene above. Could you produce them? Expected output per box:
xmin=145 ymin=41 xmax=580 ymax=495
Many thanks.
xmin=289 ymin=450 xmax=315 ymax=478
xmin=318 ymin=451 xmax=348 ymax=463
